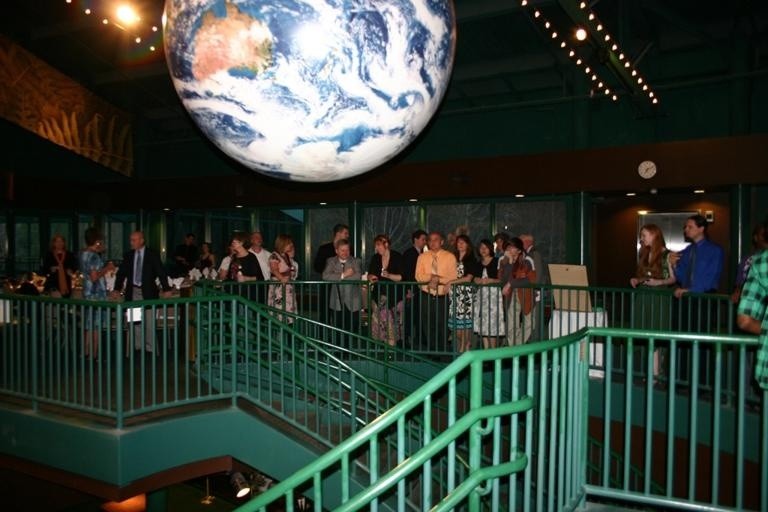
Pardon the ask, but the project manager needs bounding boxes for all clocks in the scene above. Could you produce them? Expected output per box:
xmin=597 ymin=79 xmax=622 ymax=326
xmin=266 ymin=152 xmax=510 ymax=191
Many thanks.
xmin=638 ymin=159 xmax=657 ymax=180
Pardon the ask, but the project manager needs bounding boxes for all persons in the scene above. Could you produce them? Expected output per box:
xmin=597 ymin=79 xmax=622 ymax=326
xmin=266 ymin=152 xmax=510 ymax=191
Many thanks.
xmin=629 ymin=224 xmax=678 ymax=386
xmin=729 ymin=226 xmax=766 ymax=302
xmin=738 ymin=234 xmax=768 ymax=392
xmin=39 ymin=224 xmax=546 ymax=366
xmin=667 ymin=214 xmax=721 ymax=391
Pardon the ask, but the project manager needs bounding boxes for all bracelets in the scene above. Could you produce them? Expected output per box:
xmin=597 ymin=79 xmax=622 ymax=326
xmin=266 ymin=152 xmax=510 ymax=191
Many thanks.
xmin=655 ymin=279 xmax=659 ymax=287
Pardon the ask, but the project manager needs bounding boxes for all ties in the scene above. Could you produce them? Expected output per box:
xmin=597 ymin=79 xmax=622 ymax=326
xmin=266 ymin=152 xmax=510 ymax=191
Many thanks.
xmin=135 ymin=250 xmax=142 ymax=284
xmin=432 ymin=256 xmax=438 ymax=297
